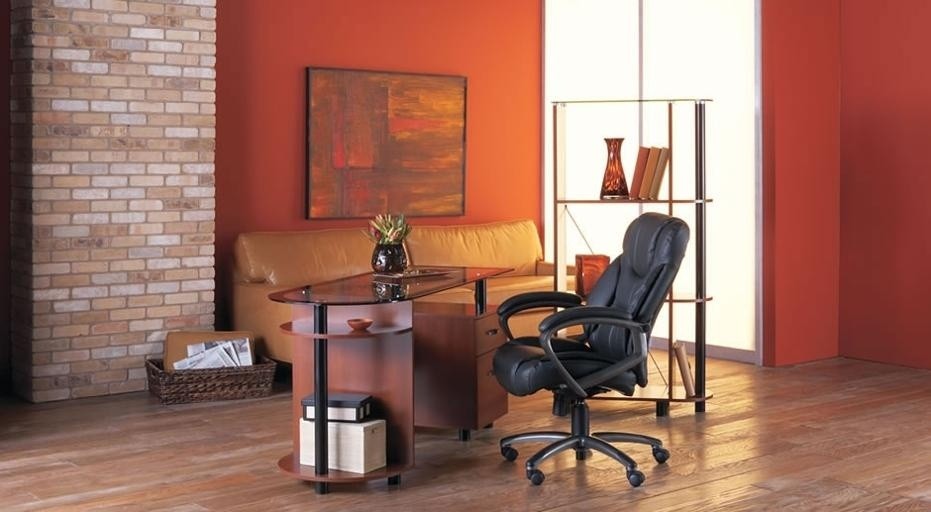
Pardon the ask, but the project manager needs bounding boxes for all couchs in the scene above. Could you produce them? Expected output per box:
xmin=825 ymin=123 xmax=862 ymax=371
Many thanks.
xmin=228 ymin=222 xmax=576 ymax=365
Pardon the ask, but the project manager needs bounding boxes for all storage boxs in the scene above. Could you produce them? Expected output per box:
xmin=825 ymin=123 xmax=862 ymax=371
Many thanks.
xmin=293 ymin=420 xmax=389 ymax=476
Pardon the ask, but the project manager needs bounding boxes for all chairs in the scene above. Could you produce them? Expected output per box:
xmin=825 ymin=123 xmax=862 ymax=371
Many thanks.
xmin=492 ymin=210 xmax=692 ymax=488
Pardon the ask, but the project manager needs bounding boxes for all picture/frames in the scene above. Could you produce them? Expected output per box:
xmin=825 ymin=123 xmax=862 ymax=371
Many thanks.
xmin=305 ymin=69 xmax=472 ymax=222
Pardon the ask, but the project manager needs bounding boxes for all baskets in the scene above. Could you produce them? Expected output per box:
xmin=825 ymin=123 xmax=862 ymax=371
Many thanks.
xmin=144 ymin=353 xmax=278 ymax=406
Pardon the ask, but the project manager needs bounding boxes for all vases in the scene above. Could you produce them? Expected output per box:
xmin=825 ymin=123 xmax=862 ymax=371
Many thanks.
xmin=602 ymin=134 xmax=626 ymax=196
xmin=363 ymin=244 xmax=414 ymax=273
xmin=371 ymin=278 xmax=409 ymax=304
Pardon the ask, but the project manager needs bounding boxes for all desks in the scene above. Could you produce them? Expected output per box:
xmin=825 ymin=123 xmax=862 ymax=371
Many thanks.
xmin=268 ymin=263 xmax=512 ymax=499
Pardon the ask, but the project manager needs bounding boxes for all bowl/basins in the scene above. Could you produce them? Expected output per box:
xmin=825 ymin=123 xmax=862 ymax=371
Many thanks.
xmin=347 ymin=318 xmax=373 ymax=330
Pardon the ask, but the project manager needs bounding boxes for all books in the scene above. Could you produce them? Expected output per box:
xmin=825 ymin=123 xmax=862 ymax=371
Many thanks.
xmin=639 ymin=146 xmax=662 ymax=199
xmin=629 ymin=144 xmax=650 ymax=198
xmin=648 ymin=147 xmax=670 ymax=200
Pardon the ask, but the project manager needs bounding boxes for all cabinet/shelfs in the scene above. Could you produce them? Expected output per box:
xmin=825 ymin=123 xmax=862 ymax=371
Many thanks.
xmin=547 ymin=98 xmax=714 ymax=419
